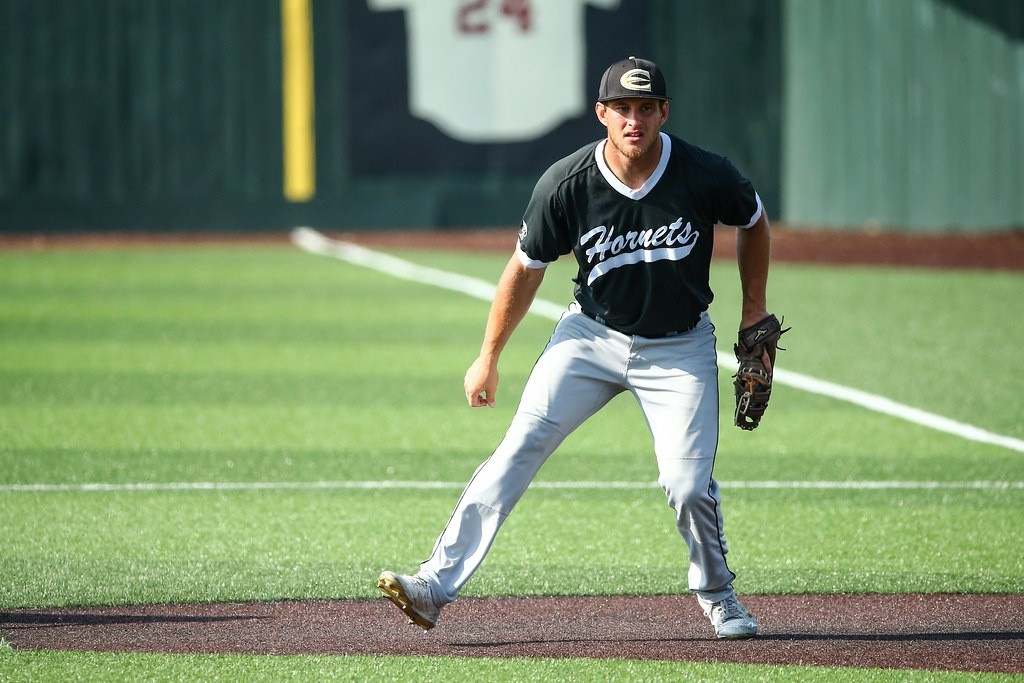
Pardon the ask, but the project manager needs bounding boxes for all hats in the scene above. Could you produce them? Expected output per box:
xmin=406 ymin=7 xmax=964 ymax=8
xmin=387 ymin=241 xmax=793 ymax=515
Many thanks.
xmin=598 ymin=56 xmax=672 ymax=104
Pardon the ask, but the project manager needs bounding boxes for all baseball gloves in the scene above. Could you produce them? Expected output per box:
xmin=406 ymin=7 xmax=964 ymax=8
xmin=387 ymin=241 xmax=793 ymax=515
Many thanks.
xmin=732 ymin=313 xmax=783 ymax=431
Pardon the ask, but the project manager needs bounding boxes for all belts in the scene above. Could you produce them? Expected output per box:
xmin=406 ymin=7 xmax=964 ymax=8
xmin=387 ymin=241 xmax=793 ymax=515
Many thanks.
xmin=580 ymin=307 xmax=701 ymax=339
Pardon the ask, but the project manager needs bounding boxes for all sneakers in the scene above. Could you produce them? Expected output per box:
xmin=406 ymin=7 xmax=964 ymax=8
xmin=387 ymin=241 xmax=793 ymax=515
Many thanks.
xmin=696 ymin=592 xmax=758 ymax=638
xmin=377 ymin=571 xmax=440 ymax=631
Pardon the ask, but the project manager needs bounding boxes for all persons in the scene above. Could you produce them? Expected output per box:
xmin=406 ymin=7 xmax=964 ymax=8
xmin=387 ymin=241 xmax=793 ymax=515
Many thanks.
xmin=377 ymin=56 xmax=771 ymax=639
